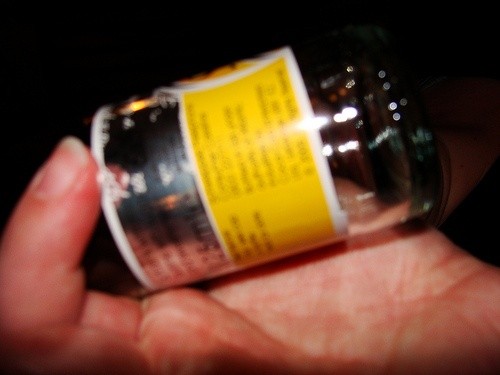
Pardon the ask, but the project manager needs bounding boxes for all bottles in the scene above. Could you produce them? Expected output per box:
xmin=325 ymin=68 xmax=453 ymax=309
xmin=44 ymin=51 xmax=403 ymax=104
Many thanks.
xmin=0 ymin=25 xmax=443 ymax=297
